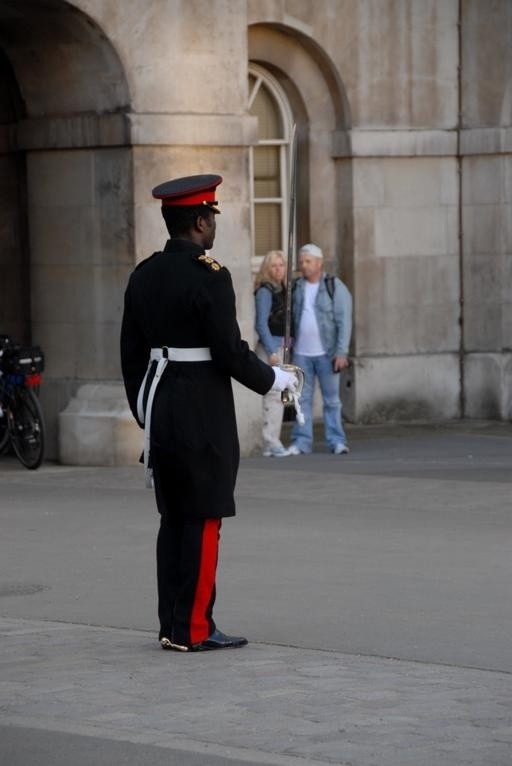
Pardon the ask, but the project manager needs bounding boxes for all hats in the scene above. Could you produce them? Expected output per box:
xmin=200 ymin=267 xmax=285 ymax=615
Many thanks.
xmin=299 ymin=244 xmax=323 ymax=258
xmin=152 ymin=175 xmax=222 ymax=215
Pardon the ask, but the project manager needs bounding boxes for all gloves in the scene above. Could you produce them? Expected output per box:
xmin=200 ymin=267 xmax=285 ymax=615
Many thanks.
xmin=271 ymin=365 xmax=297 ymax=393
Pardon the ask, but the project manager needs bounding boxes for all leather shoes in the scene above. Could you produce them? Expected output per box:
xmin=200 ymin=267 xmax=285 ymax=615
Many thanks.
xmin=161 ymin=632 xmax=247 ymax=651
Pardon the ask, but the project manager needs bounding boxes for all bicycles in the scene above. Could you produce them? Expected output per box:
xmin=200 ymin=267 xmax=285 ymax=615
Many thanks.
xmin=0 ymin=331 xmax=46 ymax=468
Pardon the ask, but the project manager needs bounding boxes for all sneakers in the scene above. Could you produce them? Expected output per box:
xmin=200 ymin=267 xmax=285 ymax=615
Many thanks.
xmin=263 ymin=444 xmax=302 ymax=458
xmin=334 ymin=444 xmax=349 ymax=454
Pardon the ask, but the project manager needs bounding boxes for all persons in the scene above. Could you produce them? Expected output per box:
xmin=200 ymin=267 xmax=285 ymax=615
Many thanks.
xmin=120 ymin=173 xmax=299 ymax=653
xmin=252 ymin=242 xmax=354 ymax=458
